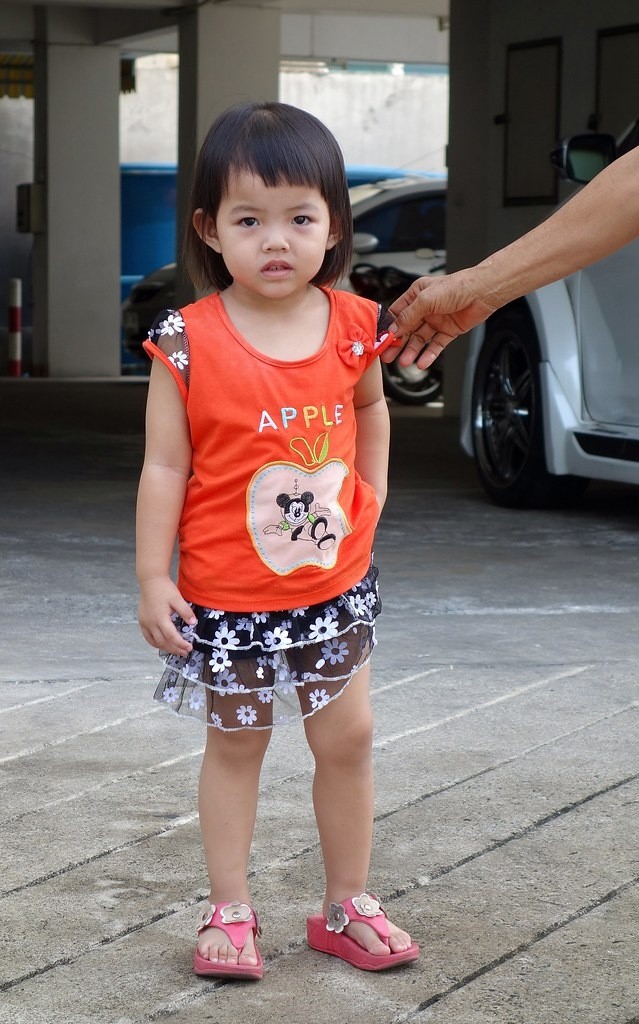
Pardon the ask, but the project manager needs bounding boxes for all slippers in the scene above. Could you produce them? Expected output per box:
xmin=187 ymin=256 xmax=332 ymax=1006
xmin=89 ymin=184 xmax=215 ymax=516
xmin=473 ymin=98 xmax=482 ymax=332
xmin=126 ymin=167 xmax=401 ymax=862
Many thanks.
xmin=307 ymin=894 xmax=419 ymax=971
xmin=194 ymin=901 xmax=264 ymax=979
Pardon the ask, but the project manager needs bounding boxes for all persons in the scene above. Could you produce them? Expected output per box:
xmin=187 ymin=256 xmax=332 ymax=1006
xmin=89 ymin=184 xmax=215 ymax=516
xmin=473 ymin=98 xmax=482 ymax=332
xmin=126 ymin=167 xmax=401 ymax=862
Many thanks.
xmin=137 ymin=99 xmax=418 ymax=981
xmin=375 ymin=144 xmax=639 ymax=370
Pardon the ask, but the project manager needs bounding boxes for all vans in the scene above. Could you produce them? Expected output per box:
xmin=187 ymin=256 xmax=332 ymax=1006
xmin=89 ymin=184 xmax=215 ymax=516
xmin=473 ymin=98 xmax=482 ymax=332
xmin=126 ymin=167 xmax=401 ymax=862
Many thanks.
xmin=23 ymin=161 xmax=443 ymax=377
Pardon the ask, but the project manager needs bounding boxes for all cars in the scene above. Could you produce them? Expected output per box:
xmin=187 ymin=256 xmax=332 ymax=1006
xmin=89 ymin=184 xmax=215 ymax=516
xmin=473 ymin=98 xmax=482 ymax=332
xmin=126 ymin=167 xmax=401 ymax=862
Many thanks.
xmin=458 ymin=115 xmax=639 ymax=507
xmin=123 ymin=174 xmax=449 ymax=404
